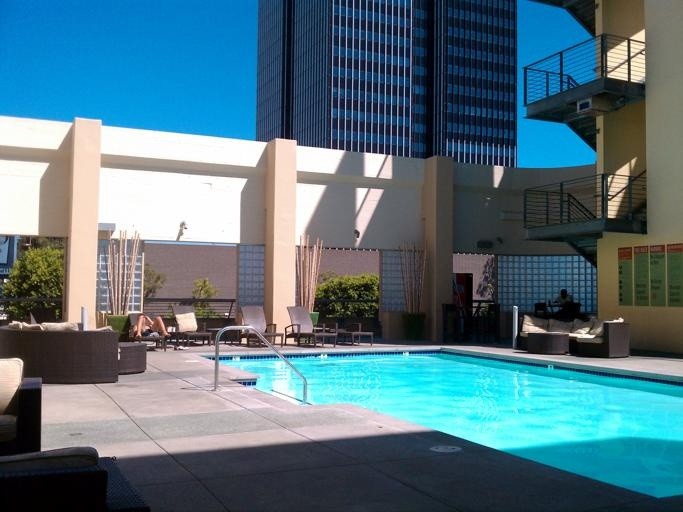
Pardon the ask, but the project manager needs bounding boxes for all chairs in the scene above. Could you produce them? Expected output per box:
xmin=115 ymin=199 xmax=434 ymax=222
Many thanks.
xmin=0 ymin=357 xmax=108 ymax=511
xmin=241 ymin=304 xmax=374 ymax=349
xmin=128 ymin=305 xmax=211 ymax=352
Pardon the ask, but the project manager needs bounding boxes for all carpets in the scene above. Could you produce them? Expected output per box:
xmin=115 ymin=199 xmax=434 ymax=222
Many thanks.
xmin=100 ymin=456 xmax=151 ymax=511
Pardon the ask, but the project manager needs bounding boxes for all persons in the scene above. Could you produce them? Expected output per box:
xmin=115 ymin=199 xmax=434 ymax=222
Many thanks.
xmin=552 ymin=288 xmax=573 ymax=309
xmin=131 ymin=313 xmax=171 ymax=340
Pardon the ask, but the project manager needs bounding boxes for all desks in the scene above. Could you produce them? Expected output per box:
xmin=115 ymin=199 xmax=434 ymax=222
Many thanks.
xmin=207 ymin=327 xmax=237 ymax=346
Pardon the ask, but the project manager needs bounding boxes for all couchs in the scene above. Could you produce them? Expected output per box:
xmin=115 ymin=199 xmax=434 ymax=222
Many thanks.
xmin=516 ymin=312 xmax=631 ymax=359
xmin=0 ymin=323 xmax=118 ymax=384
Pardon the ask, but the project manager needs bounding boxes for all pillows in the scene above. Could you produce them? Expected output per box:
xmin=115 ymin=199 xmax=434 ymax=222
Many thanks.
xmin=8 ymin=321 xmax=79 ymax=331
xmin=521 ymin=314 xmax=609 ymax=335
xmin=0 ymin=358 xmax=24 ymax=415
